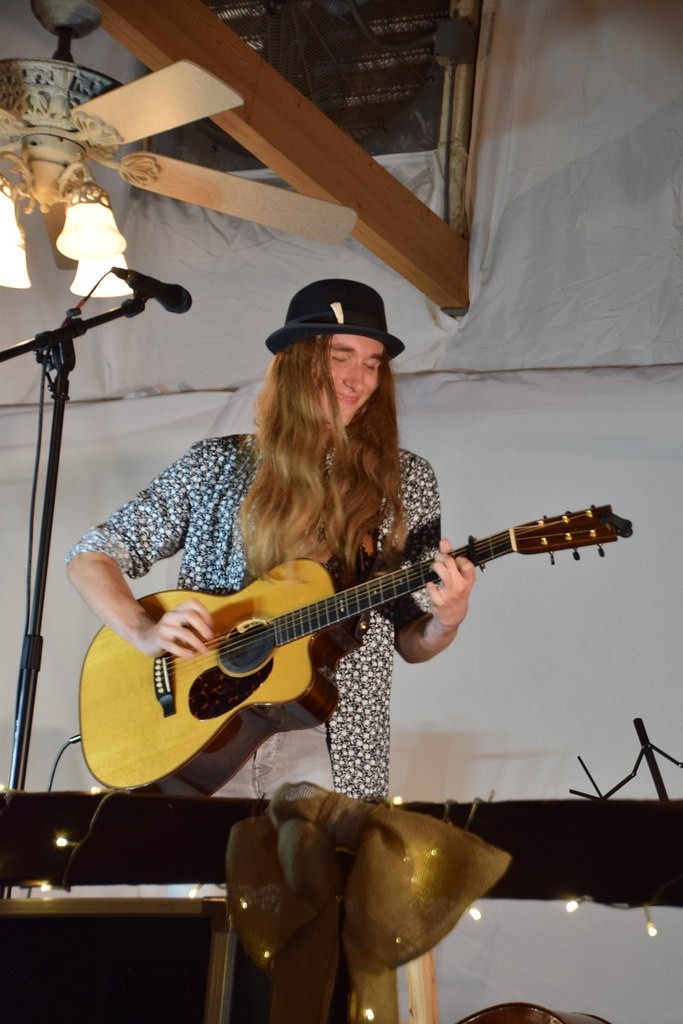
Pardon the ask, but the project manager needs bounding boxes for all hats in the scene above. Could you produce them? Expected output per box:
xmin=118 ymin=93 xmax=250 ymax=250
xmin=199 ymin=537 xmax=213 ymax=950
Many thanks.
xmin=265 ymin=279 xmax=405 ymax=361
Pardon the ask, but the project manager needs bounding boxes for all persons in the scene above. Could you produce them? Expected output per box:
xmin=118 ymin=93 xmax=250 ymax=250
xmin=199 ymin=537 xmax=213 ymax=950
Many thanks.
xmin=65 ymin=278 xmax=475 ymax=798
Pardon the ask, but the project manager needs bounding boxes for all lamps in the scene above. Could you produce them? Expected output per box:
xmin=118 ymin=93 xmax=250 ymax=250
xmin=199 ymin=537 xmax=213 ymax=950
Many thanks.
xmin=0 ymin=152 xmax=135 ymax=299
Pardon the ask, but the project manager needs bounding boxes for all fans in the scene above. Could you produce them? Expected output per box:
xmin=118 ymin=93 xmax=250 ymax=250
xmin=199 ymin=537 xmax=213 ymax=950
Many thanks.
xmin=0 ymin=0 xmax=358 ymax=271
xmin=131 ymin=1 xmax=455 ymax=159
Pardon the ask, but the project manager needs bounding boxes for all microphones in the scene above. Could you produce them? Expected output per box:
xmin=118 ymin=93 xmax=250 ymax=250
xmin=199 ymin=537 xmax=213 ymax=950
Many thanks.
xmin=112 ymin=267 xmax=193 ymax=314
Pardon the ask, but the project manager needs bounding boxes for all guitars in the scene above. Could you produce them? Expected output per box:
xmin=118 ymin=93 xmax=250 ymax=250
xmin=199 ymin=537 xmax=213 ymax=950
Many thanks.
xmin=77 ymin=502 xmax=634 ymax=798
